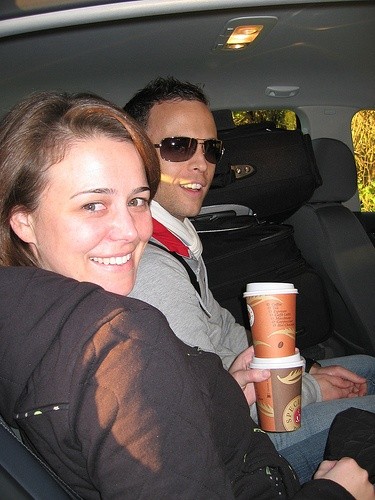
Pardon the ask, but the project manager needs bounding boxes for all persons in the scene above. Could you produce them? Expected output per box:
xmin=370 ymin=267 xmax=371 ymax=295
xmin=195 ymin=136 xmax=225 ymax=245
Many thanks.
xmin=0 ymin=90 xmax=375 ymax=500
xmin=120 ymin=77 xmax=375 ymax=449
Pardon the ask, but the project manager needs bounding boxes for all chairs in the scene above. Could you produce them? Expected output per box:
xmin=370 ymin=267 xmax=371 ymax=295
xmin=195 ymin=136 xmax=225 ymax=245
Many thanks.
xmin=282 ymin=139 xmax=375 ymax=355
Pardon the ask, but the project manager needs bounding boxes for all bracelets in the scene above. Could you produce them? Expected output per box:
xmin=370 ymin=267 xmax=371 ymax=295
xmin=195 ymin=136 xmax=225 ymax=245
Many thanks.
xmin=304 ymin=360 xmax=320 ymax=374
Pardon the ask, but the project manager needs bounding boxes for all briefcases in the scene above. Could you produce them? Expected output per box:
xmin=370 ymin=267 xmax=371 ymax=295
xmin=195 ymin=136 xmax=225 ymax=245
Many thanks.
xmin=201 ymin=122 xmax=323 ymax=225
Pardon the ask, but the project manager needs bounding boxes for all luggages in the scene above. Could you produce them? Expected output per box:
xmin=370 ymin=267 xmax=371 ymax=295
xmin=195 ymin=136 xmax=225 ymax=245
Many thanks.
xmin=189 ymin=210 xmax=331 ymax=352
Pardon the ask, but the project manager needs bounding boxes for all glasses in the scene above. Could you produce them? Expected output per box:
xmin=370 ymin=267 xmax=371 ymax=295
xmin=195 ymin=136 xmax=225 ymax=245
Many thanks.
xmin=153 ymin=137 xmax=225 ymax=164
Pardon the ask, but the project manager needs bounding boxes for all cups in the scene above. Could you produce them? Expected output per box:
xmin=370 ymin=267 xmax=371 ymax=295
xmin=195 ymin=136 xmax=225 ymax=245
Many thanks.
xmin=248 ymin=353 xmax=305 ymax=432
xmin=243 ymin=283 xmax=298 ymax=358
